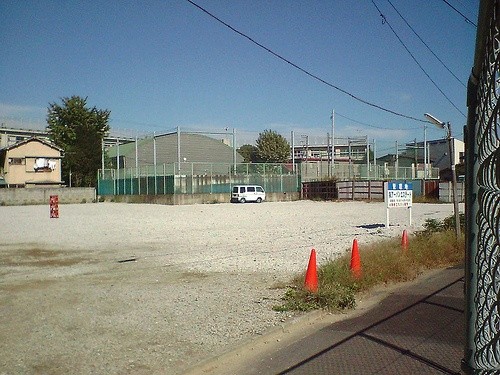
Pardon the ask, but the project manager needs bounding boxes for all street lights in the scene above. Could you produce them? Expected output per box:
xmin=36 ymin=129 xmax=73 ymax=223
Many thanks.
xmin=424 ymin=113 xmax=461 ymax=243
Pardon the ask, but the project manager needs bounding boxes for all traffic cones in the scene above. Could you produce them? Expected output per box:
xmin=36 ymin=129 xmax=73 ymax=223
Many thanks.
xmin=401 ymin=230 xmax=410 ymax=251
xmin=351 ymin=239 xmax=362 ymax=281
xmin=303 ymin=248 xmax=321 ymax=301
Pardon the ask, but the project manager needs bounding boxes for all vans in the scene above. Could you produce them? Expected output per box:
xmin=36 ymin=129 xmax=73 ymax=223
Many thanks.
xmin=231 ymin=184 xmax=266 ymax=203
